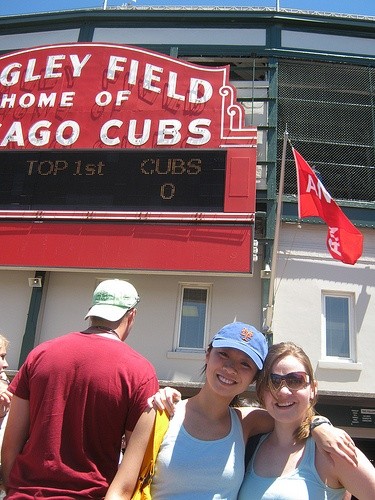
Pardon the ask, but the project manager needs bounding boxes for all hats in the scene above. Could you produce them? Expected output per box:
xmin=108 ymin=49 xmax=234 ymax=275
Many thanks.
xmin=209 ymin=323 xmax=269 ymax=370
xmin=84 ymin=278 xmax=140 ymax=322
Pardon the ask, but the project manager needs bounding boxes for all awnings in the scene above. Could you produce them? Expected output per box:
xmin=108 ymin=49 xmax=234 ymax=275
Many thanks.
xmin=315 ymin=403 xmax=375 ymax=438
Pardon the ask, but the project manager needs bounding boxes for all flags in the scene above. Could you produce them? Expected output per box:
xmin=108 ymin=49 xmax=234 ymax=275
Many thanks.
xmin=292 ymin=146 xmax=364 ymax=265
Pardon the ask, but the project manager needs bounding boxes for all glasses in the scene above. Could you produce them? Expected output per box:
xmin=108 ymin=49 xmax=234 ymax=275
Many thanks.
xmin=262 ymin=371 xmax=311 ymax=392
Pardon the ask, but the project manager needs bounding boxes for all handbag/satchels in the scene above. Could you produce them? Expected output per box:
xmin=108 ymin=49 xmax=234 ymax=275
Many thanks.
xmin=131 ymin=403 xmax=170 ymax=500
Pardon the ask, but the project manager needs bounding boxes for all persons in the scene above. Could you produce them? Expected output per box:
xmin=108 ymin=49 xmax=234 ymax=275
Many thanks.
xmin=146 ymin=340 xmax=375 ymax=500
xmin=103 ymin=322 xmax=359 ymax=500
xmin=118 ymin=437 xmax=126 ymax=464
xmin=0 ymin=335 xmax=13 ymax=428
xmin=0 ymin=279 xmax=158 ymax=500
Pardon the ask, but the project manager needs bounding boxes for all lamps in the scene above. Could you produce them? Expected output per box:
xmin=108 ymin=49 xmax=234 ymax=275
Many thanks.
xmin=27 ymin=276 xmax=43 ymax=288
xmin=261 ymin=264 xmax=271 ymax=279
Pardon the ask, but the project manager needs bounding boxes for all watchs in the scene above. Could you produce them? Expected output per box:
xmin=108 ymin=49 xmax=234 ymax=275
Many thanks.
xmin=311 ymin=416 xmax=332 ymax=431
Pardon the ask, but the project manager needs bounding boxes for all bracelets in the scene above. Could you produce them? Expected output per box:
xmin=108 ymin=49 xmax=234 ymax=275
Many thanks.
xmin=0 ymin=413 xmax=6 ymax=418
